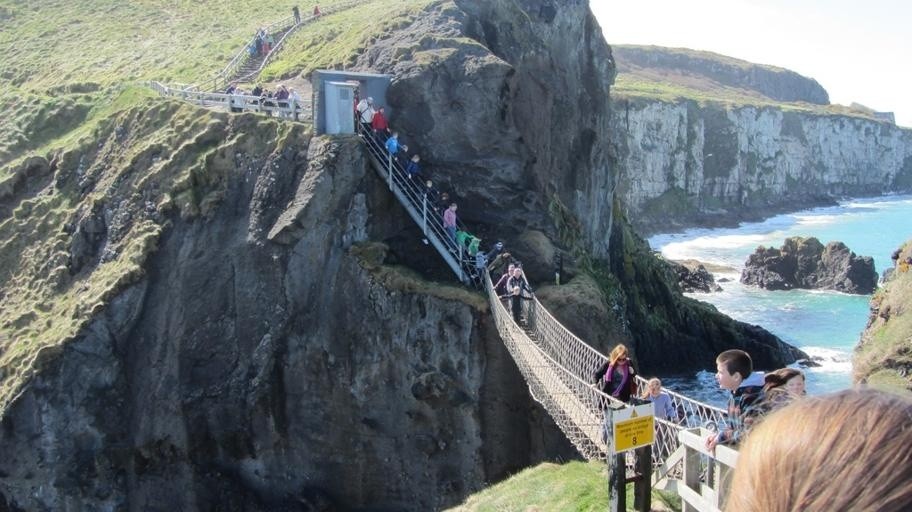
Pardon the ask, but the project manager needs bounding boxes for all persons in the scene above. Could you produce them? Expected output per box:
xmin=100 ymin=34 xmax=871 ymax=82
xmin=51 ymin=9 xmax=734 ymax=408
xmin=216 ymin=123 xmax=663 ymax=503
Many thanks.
xmin=292 ymin=5 xmax=300 ymax=25
xmin=590 ymin=342 xmax=638 ymax=445
xmin=248 ymin=27 xmax=274 ymax=57
xmin=225 ymin=81 xmax=536 ymax=325
xmin=640 ymin=376 xmax=676 ymax=463
xmin=764 ymin=368 xmax=806 ymax=411
xmin=723 ymin=387 xmax=912 ymax=512
xmin=705 ymin=349 xmax=770 ymax=456
xmin=314 ymin=6 xmax=321 ymax=19
